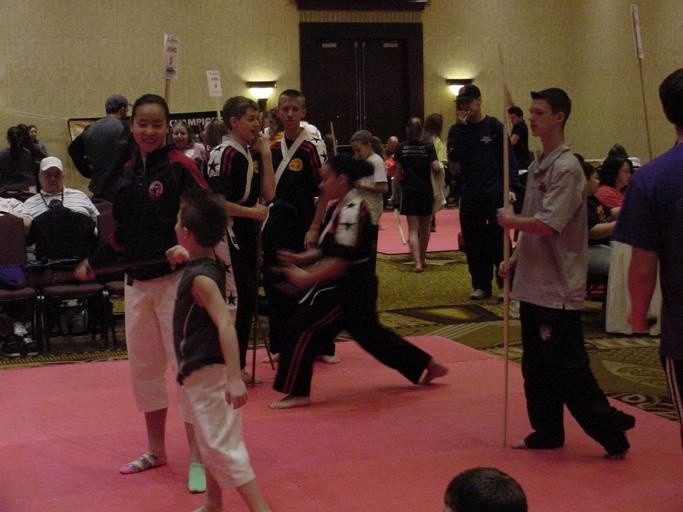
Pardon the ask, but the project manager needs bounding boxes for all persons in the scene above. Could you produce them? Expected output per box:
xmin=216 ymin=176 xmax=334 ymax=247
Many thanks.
xmin=67 ymin=94 xmax=131 ymax=198
xmin=201 ymin=96 xmax=275 ymax=384
xmin=352 ymin=130 xmax=398 ymax=259
xmin=256 ymin=89 xmax=341 ymax=363
xmin=496 ymin=87 xmax=636 ymax=459
xmin=448 ymin=85 xmax=518 ymax=298
xmin=573 ymin=145 xmax=634 ymax=273
xmin=445 ymin=467 xmax=529 ymax=511
xmin=2 ymin=198 xmax=39 ymax=357
xmin=269 ymin=108 xmax=278 ymax=127
xmin=168 ymin=118 xmax=225 ymax=174
xmin=174 ymin=201 xmax=270 ymax=509
xmin=391 ymin=114 xmax=447 ymax=273
xmin=507 ymin=106 xmax=530 ymax=186
xmin=613 ymin=68 xmax=682 ymax=398
xmin=269 ymin=153 xmax=449 ymax=407
xmin=16 ymin=157 xmax=101 ymax=335
xmin=2 ymin=124 xmax=48 ymax=199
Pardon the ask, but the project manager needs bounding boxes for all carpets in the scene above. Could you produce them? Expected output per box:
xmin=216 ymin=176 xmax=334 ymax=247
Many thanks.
xmin=0 ymin=333 xmax=683 ymax=511
xmin=375 ymin=207 xmax=519 ymax=256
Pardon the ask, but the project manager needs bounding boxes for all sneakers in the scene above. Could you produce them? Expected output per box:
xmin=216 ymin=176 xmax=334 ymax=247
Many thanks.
xmin=22 ymin=334 xmax=39 ymax=356
xmin=469 ymin=289 xmax=485 ymax=299
xmin=0 ymin=335 xmax=21 ymax=357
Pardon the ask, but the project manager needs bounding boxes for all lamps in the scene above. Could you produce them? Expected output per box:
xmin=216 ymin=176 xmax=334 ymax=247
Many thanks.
xmin=246 ymin=80 xmax=277 ymax=111
xmin=445 ymin=78 xmax=473 ymax=97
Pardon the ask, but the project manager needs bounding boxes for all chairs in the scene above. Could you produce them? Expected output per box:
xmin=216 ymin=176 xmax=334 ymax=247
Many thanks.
xmin=0 ymin=210 xmax=41 ymax=357
xmin=88 ymin=195 xmax=112 ymax=210
xmin=30 ymin=210 xmax=118 ymax=354
xmin=95 ymin=210 xmax=126 ymax=350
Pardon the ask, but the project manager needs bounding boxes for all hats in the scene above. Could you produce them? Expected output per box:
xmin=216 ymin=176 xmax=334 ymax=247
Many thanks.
xmin=40 ymin=157 xmax=62 ymax=173
xmin=454 ymin=85 xmax=480 ymax=102
xmin=106 ymin=95 xmax=131 ymax=111
